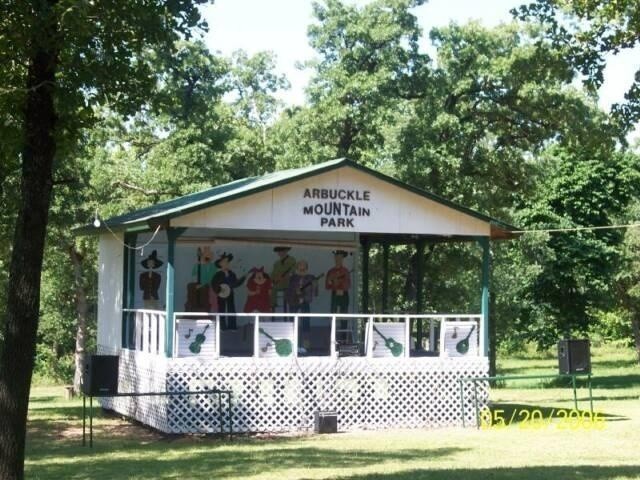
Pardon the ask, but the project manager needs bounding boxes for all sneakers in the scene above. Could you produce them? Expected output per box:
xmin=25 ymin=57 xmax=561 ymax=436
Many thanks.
xmin=219 ymin=327 xmax=241 ymax=333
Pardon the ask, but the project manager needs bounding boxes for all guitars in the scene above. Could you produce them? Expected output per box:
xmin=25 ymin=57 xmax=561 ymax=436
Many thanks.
xmin=372 ymin=325 xmax=403 ymax=357
xmin=188 ymin=324 xmax=209 ymax=354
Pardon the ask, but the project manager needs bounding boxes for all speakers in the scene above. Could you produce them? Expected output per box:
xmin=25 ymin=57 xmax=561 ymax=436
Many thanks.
xmin=82 ymin=354 xmax=119 ymax=396
xmin=558 ymin=338 xmax=591 ymax=375
xmin=314 ymin=411 xmax=337 ymax=434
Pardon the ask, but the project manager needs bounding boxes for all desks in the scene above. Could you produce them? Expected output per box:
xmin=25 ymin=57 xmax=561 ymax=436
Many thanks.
xmin=83 ymin=390 xmax=233 ymax=449
xmin=457 ymin=373 xmax=593 ymax=430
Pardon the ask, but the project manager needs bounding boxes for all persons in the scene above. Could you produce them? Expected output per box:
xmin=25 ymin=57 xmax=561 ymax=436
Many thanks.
xmin=139 ymin=249 xmax=164 ymax=309
xmin=186 ymin=246 xmax=349 ymax=354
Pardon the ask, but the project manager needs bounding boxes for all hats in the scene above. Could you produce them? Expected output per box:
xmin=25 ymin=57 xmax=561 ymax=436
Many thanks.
xmin=331 ymin=249 xmax=348 ymax=258
xmin=141 ymin=249 xmax=164 ymax=269
xmin=215 ymin=251 xmax=234 ymax=269
xmin=272 ymin=247 xmax=292 ymax=252
